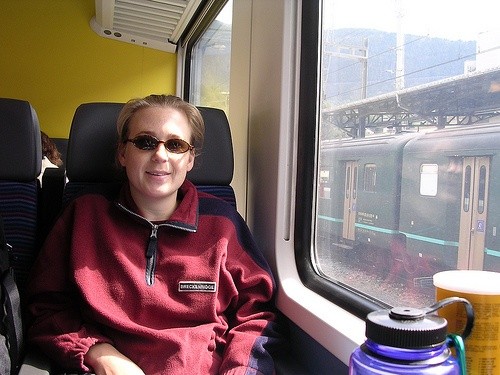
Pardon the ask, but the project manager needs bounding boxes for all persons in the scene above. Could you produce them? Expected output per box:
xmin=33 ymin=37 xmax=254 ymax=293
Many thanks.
xmin=24 ymin=94 xmax=277 ymax=375
xmin=36 ymin=131 xmax=69 ymax=190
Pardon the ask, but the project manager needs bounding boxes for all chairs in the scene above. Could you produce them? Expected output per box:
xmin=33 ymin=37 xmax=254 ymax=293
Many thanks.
xmin=0 ymin=98 xmax=303 ymax=375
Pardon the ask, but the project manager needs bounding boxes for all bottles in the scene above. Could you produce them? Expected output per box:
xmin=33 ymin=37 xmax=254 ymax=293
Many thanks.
xmin=349 ymin=297 xmax=474 ymax=375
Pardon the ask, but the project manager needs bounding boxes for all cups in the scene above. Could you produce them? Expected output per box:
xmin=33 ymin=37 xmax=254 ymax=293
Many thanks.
xmin=433 ymin=270 xmax=500 ymax=375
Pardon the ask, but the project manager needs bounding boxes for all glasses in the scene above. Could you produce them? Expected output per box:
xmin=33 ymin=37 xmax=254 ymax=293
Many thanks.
xmin=126 ymin=135 xmax=193 ymax=154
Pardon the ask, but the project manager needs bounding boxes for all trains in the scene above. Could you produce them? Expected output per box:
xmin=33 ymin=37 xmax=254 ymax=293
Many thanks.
xmin=316 ymin=122 xmax=500 ymax=277
